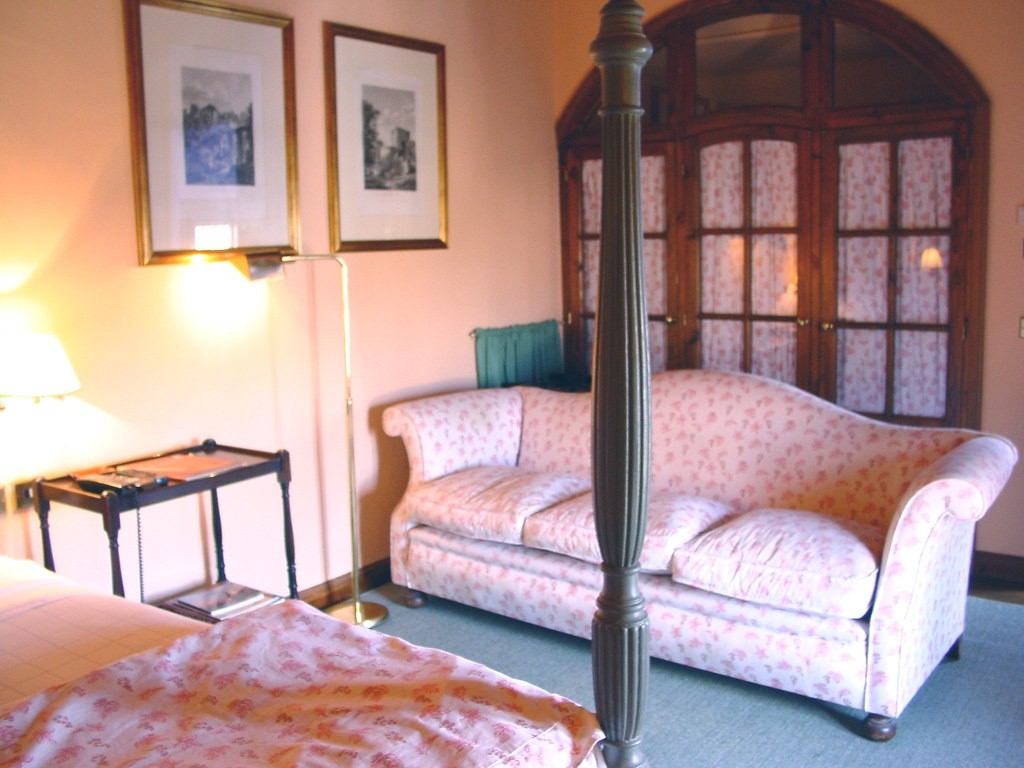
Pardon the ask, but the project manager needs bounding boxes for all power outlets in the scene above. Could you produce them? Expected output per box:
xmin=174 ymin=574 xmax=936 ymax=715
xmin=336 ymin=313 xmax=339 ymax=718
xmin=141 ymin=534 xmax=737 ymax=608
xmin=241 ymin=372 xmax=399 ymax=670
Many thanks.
xmin=12 ymin=479 xmax=37 ymax=511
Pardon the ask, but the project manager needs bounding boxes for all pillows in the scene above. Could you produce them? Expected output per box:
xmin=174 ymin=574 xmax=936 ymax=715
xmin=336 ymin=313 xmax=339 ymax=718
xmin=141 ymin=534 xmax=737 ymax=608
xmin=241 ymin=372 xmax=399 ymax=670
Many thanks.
xmin=671 ymin=506 xmax=888 ymax=619
xmin=407 ymin=465 xmax=588 ymax=545
xmin=521 ymin=486 xmax=743 ymax=576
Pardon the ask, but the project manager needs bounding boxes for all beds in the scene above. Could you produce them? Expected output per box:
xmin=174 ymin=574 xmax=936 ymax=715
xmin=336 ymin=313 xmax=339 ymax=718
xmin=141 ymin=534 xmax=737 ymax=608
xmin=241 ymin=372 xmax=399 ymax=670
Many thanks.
xmin=0 ymin=552 xmax=653 ymax=768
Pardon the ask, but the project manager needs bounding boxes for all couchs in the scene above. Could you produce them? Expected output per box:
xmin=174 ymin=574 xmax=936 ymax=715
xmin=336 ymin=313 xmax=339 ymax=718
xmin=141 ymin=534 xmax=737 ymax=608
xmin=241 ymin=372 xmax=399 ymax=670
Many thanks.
xmin=381 ymin=368 xmax=1018 ymax=741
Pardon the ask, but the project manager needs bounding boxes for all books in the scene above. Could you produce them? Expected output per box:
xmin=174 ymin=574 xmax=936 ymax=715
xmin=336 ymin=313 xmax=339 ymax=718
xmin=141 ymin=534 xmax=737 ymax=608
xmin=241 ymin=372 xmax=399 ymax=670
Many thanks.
xmin=162 ymin=580 xmax=286 ymax=624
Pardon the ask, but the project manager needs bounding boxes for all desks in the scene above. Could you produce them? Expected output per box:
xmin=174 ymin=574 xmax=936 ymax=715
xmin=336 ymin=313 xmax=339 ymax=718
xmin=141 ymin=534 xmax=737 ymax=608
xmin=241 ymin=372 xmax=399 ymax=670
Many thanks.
xmin=29 ymin=438 xmax=299 ymax=624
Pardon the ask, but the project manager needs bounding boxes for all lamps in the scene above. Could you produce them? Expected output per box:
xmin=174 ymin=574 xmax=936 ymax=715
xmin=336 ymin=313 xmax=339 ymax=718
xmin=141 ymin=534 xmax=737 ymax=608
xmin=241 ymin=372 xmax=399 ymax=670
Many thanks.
xmin=230 ymin=248 xmax=389 ymax=630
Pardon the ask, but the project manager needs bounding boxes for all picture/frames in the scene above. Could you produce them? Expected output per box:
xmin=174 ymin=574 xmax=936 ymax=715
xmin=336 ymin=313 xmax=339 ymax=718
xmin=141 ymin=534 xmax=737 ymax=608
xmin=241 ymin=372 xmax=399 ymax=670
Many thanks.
xmin=322 ymin=20 xmax=450 ymax=253
xmin=123 ymin=0 xmax=301 ymax=267
